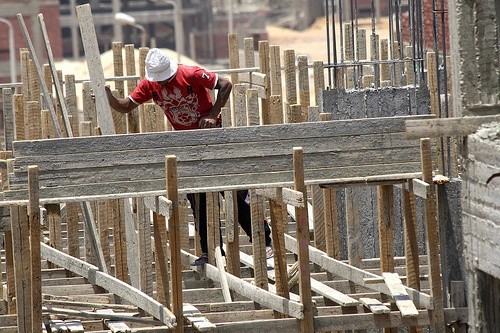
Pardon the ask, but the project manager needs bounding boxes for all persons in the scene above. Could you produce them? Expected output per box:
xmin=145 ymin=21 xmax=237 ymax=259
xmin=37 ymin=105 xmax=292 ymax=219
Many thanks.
xmin=90 ymin=47 xmax=275 ymax=267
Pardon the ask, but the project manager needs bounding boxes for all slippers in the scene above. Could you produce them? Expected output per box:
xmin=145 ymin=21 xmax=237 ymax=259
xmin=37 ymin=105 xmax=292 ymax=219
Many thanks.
xmin=191 ymin=253 xmax=208 ymax=265
xmin=266 ymin=247 xmax=273 ymax=259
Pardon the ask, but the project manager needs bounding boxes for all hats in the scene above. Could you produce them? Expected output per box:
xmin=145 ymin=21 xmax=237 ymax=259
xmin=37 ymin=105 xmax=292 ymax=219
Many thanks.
xmin=144 ymin=48 xmax=178 ymax=82
